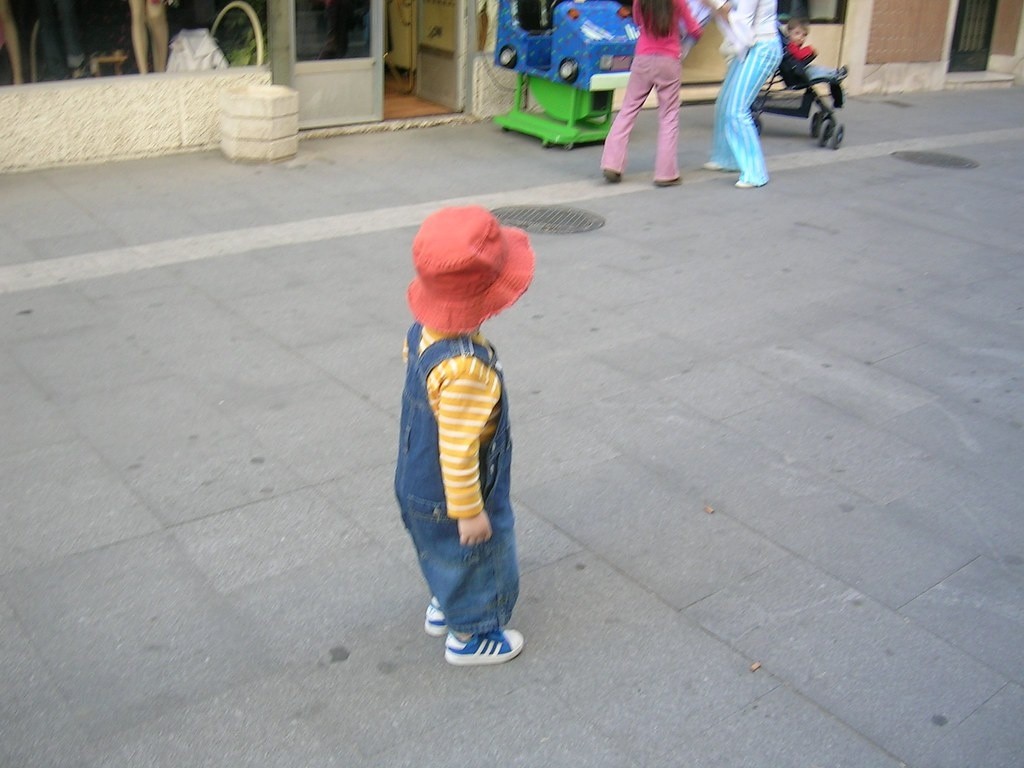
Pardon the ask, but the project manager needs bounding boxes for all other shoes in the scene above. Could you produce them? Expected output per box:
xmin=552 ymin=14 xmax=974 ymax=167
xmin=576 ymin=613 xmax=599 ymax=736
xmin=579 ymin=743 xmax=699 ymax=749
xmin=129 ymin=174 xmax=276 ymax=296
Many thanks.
xmin=835 ymin=67 xmax=847 ymax=84
xmin=654 ymin=173 xmax=678 ymax=186
xmin=735 ymin=180 xmax=754 ymax=188
xmin=705 ymin=161 xmax=724 ymax=171
xmin=603 ymin=169 xmax=621 ymax=183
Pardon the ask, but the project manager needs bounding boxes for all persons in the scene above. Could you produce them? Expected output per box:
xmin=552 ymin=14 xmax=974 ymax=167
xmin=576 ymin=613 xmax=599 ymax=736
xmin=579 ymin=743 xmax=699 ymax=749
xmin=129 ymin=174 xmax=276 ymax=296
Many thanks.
xmin=704 ymin=0 xmax=784 ymax=189
xmin=393 ymin=204 xmax=534 ymax=666
xmin=786 ymin=17 xmax=849 ymax=83
xmin=600 ymin=0 xmax=703 ymax=188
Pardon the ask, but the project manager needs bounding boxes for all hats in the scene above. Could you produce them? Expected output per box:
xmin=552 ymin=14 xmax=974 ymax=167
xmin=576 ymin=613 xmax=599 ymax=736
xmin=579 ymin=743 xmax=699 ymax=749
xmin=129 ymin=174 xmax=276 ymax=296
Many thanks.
xmin=407 ymin=205 xmax=533 ymax=334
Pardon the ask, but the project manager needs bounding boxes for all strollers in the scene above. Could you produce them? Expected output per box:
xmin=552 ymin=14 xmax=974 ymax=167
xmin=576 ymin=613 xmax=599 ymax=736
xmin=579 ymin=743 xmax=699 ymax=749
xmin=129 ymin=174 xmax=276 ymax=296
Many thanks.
xmin=751 ymin=22 xmax=847 ymax=151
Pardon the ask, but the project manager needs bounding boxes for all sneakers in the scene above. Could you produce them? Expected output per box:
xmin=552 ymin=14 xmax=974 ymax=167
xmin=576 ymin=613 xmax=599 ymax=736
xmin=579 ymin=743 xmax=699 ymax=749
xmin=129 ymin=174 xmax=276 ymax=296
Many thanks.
xmin=444 ymin=629 xmax=524 ymax=666
xmin=424 ymin=596 xmax=449 ymax=637
